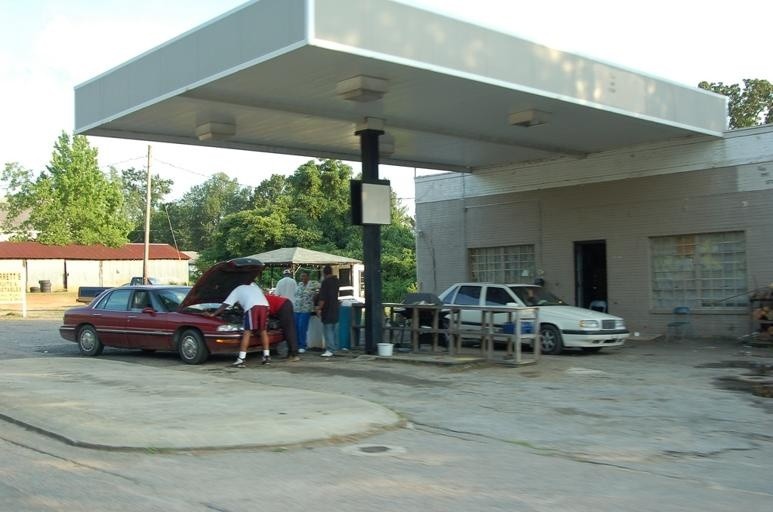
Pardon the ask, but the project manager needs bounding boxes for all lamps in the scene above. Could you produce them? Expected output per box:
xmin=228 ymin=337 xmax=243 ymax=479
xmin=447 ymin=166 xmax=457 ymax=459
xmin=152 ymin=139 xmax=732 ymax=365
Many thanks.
xmin=377 ymin=127 xmax=396 ymax=156
xmin=194 ymin=120 xmax=239 ymax=144
xmin=336 ymin=71 xmax=397 ymax=111
xmin=505 ymin=106 xmax=556 ymax=130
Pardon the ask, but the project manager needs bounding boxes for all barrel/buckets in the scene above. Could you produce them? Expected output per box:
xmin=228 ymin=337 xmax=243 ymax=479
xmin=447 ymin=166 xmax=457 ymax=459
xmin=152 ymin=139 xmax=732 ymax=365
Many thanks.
xmin=378 ymin=343 xmax=394 ymax=357
xmin=338 ymin=299 xmax=362 ymax=348
xmin=352 ymin=303 xmax=366 ymax=350
xmin=306 ymin=312 xmax=326 ymax=348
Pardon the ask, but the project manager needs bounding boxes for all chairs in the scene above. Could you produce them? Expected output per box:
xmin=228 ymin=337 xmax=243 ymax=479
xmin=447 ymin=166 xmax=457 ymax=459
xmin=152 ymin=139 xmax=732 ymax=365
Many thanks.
xmin=587 ymin=299 xmax=608 ymax=316
xmin=666 ymin=305 xmax=691 ymax=341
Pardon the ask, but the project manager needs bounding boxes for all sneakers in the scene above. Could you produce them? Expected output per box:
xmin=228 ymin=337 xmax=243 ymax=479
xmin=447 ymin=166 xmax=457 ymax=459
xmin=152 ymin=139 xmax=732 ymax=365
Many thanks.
xmin=281 ymin=354 xmax=300 ymax=363
xmin=261 ymin=355 xmax=272 ymax=366
xmin=320 ymin=350 xmax=334 ymax=357
xmin=232 ymin=357 xmax=246 ymax=368
xmin=341 ymin=348 xmax=349 ymax=351
xmin=298 ymin=348 xmax=305 ymax=353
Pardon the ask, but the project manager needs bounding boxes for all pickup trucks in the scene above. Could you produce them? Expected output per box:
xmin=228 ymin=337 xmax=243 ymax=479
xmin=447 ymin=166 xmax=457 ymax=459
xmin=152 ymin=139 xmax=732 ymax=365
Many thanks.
xmin=76 ymin=276 xmax=186 ymax=309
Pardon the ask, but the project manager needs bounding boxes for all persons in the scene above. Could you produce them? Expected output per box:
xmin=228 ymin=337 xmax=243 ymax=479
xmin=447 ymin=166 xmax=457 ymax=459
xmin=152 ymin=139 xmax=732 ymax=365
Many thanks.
xmin=293 ymin=272 xmax=320 ymax=355
xmin=274 ymin=269 xmax=298 ymax=316
xmin=314 ymin=266 xmax=340 ymax=354
xmin=522 ymin=287 xmax=536 ymax=305
xmin=201 ymin=280 xmax=272 ymax=368
xmin=264 ymin=293 xmax=301 ymax=362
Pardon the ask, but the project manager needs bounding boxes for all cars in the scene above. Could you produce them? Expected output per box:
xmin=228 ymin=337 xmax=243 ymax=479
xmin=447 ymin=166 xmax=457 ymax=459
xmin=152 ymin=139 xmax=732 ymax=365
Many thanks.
xmin=57 ymin=258 xmax=288 ymax=365
xmin=435 ymin=279 xmax=630 ymax=354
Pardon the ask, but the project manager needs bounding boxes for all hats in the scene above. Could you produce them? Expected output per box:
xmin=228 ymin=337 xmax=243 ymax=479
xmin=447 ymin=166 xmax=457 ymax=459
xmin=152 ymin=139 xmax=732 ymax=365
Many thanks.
xmin=283 ymin=270 xmax=291 ymax=274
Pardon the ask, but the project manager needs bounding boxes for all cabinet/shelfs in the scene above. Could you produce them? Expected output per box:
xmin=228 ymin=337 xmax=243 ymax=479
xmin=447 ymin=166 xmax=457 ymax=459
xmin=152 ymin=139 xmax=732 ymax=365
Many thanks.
xmin=745 ymin=295 xmax=772 ymax=348
